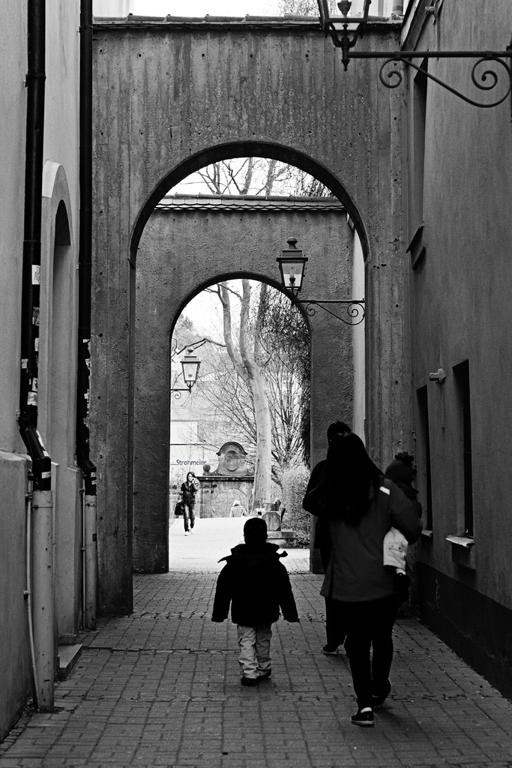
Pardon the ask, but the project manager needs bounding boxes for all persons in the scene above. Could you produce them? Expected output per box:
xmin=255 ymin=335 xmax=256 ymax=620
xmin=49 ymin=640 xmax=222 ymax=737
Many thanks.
xmin=309 ymin=422 xmax=363 ymax=657
xmin=380 ymin=451 xmax=422 ymax=605
xmin=212 ymin=518 xmax=298 ymax=686
xmin=303 ymin=434 xmax=423 ymax=728
xmin=177 ymin=472 xmax=200 ymax=536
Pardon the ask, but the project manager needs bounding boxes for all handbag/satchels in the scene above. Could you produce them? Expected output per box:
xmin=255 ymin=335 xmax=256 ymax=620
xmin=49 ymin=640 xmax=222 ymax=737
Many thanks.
xmin=175 ymin=502 xmax=184 ymax=515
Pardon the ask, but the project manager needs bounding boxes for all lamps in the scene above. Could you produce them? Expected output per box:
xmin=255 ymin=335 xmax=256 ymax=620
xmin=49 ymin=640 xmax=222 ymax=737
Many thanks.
xmin=315 ymin=1 xmax=511 ymax=114
xmin=171 ymin=344 xmax=201 ymax=393
xmin=274 ymin=236 xmax=365 ymax=326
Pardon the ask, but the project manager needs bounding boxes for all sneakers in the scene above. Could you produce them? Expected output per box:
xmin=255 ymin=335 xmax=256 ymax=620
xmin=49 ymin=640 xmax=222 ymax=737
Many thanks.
xmin=351 ymin=711 xmax=374 ymax=725
